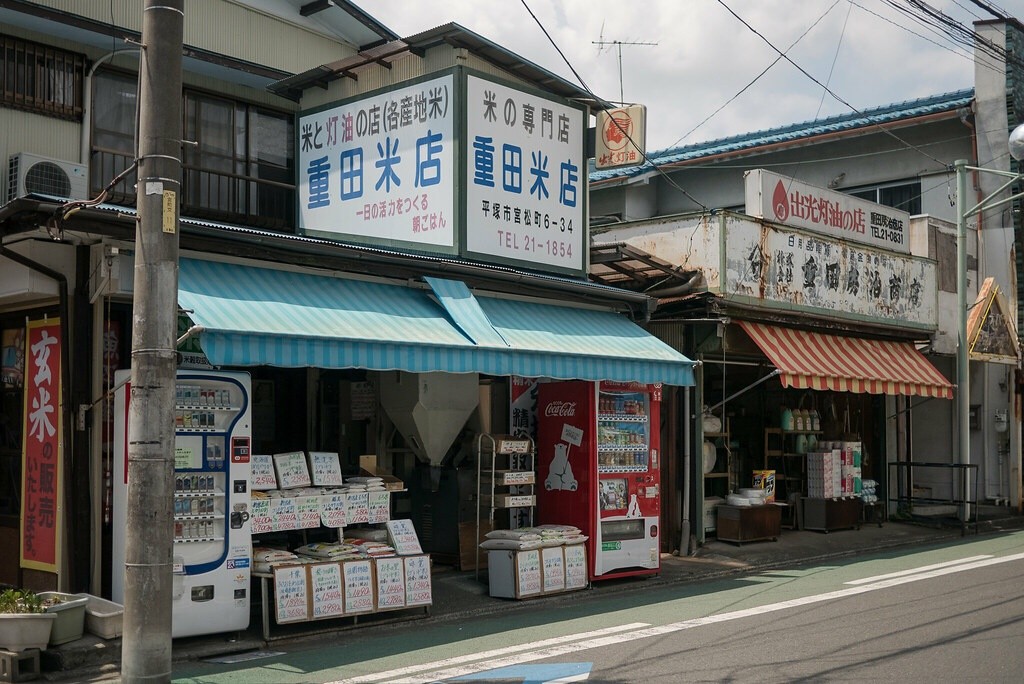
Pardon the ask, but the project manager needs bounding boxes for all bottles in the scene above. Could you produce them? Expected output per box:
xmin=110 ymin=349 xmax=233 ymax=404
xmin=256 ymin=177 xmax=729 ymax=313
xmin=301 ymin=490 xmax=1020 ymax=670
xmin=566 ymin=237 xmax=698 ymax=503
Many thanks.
xmin=809 ymin=435 xmax=818 ymax=452
xmin=781 ymin=408 xmax=794 ymax=430
xmin=600 ymin=453 xmax=644 ymax=465
xmin=616 ymin=496 xmax=628 ymax=509
xmin=599 ymin=397 xmax=645 ymax=415
xmin=598 ymin=421 xmax=644 ymax=444
xmin=795 ymin=409 xmax=805 ymax=430
xmin=176 ymin=385 xmax=229 ymax=406
xmin=801 ymin=410 xmax=812 ymax=431
xmin=796 ymin=435 xmax=807 ymax=453
xmin=176 ymin=410 xmax=214 ymax=428
xmin=810 ymin=410 xmax=820 ymax=430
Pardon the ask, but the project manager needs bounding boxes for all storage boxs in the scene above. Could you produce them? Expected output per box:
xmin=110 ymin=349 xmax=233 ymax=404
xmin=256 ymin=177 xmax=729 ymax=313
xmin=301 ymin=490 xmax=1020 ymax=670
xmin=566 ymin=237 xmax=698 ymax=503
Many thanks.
xmin=808 ymin=441 xmax=861 ymax=500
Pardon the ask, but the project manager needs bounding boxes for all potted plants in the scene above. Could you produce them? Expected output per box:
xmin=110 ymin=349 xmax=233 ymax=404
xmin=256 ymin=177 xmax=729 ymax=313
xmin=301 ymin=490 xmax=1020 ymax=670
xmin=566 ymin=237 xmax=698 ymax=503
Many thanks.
xmin=0 ymin=586 xmax=57 ymax=651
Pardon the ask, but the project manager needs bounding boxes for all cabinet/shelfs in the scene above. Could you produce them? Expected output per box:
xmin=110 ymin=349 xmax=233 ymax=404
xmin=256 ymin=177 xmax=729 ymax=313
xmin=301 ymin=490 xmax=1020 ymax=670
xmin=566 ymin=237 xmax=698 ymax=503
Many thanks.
xmin=487 ymin=545 xmax=590 ymax=600
xmin=703 ymin=418 xmax=731 ymax=543
xmin=251 ymin=488 xmax=433 ymax=642
xmin=713 ymin=504 xmax=783 ymax=545
xmin=475 ymin=433 xmax=535 ymax=581
xmin=763 ymin=427 xmax=823 ymax=500
xmin=799 ymin=496 xmax=860 ymax=535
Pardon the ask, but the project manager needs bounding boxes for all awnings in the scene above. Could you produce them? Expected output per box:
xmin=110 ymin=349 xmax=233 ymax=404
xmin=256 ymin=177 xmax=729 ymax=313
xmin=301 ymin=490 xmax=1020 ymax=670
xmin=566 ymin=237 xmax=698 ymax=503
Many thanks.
xmin=177 ymin=248 xmax=702 ymax=386
xmin=732 ymin=320 xmax=958 ymax=400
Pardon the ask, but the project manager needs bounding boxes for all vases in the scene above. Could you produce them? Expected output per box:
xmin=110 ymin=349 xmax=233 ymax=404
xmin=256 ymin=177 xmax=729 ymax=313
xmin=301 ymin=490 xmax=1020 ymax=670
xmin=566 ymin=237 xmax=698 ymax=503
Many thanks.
xmin=73 ymin=594 xmax=124 ymax=640
xmin=15 ymin=591 xmax=87 ymax=646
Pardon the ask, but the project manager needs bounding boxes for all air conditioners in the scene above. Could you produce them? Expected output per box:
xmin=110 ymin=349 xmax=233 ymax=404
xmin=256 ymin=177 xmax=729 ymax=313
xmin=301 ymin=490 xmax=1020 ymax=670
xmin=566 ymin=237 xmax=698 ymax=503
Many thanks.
xmin=2 ymin=152 xmax=90 ymax=205
